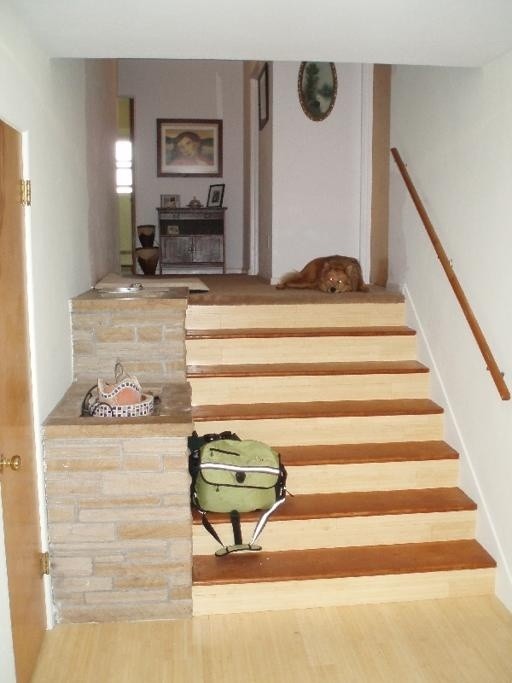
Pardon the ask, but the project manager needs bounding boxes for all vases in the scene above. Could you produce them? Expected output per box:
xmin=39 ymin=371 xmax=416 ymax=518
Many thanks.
xmin=134 ymin=224 xmax=159 ymax=275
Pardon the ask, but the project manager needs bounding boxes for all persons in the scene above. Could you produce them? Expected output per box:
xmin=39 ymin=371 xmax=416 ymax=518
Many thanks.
xmin=168 ymin=130 xmax=214 ymax=166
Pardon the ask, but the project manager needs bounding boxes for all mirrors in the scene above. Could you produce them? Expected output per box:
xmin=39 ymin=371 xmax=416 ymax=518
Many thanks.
xmin=297 ymin=61 xmax=338 ymax=121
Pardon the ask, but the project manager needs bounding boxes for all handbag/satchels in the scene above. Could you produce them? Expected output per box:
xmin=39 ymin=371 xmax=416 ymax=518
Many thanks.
xmin=196 ymin=439 xmax=286 ymax=516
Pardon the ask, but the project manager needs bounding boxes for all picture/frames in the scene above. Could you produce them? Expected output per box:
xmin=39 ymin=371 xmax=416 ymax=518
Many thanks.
xmin=257 ymin=62 xmax=270 ymax=129
xmin=205 ymin=184 xmax=225 ymax=206
xmin=160 ymin=194 xmax=181 ymax=208
xmin=155 ymin=118 xmax=223 ymax=177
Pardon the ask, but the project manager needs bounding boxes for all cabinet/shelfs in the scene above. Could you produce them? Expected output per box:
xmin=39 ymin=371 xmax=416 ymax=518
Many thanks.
xmin=157 ymin=207 xmax=227 ymax=274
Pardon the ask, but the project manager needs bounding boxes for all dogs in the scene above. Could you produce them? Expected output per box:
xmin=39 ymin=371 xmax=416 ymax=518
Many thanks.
xmin=276 ymin=255 xmax=369 ymax=293
